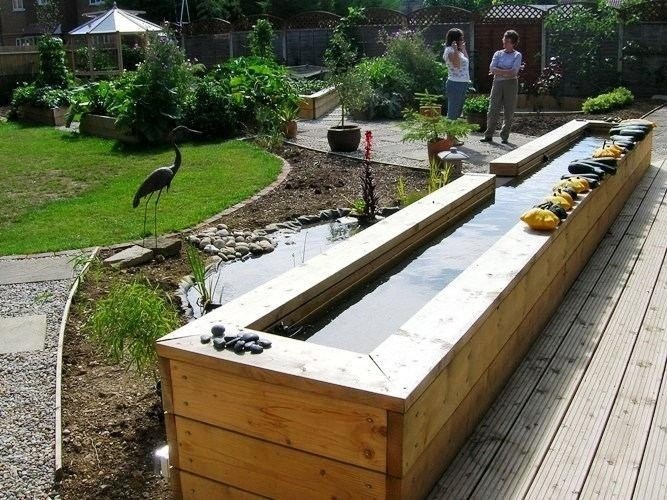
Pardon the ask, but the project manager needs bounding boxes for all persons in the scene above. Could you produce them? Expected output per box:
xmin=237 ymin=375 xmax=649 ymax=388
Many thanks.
xmin=442 ymin=28 xmax=470 ymax=147
xmin=479 ymin=29 xmax=523 ymax=144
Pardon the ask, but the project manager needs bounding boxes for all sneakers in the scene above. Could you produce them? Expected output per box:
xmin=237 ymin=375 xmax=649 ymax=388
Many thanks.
xmin=479 ymin=138 xmax=508 ymax=144
xmin=447 ymin=135 xmax=464 ymax=146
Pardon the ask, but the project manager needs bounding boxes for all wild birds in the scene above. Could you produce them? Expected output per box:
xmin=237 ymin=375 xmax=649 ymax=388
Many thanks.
xmin=133 ymin=125 xmax=203 ymax=258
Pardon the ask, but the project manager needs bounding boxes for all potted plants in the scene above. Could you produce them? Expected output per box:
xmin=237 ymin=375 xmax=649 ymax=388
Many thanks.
xmin=276 ymin=7 xmax=491 ymax=170
xmin=3 ymin=82 xmax=137 ymax=142
